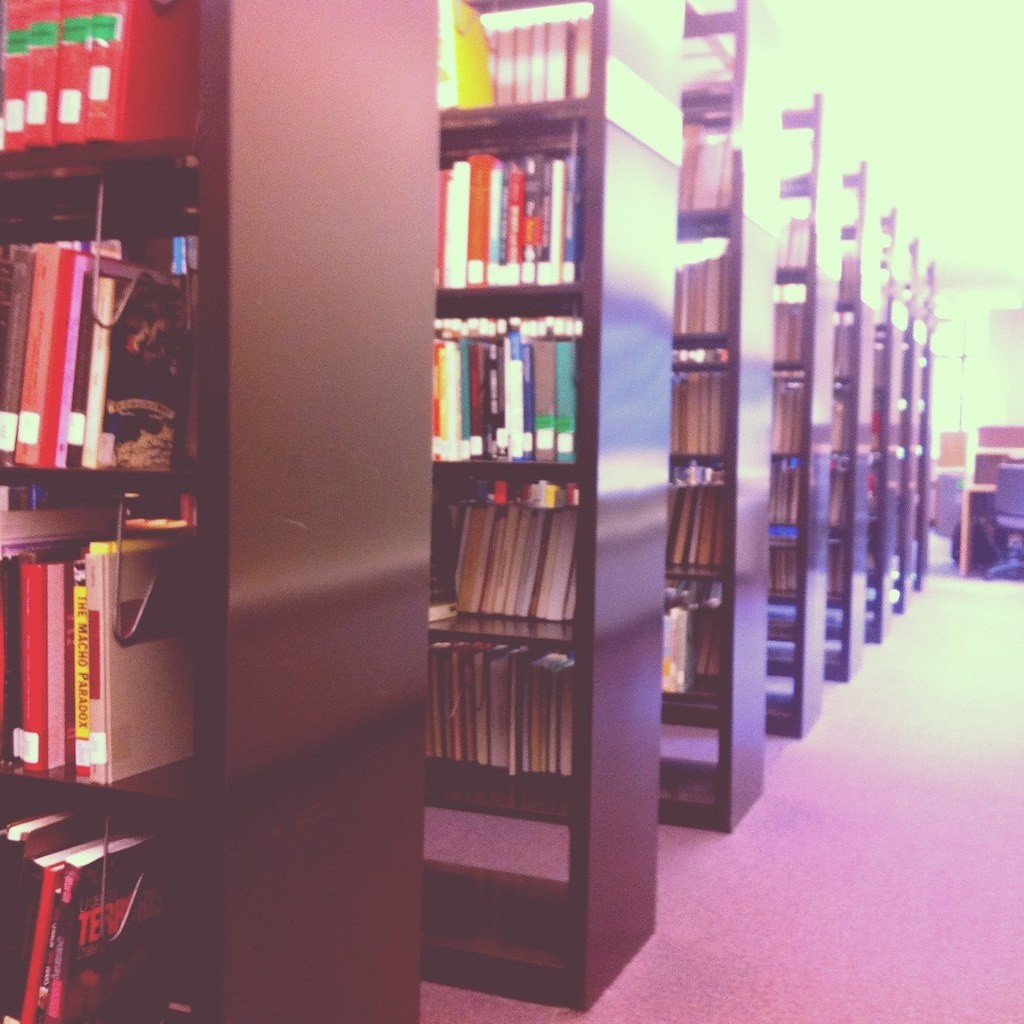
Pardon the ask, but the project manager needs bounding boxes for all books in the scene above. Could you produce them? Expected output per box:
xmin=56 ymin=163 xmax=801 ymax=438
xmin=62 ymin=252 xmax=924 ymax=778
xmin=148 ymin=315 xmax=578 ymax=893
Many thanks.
xmin=766 ymin=218 xmax=799 ymax=708
xmin=0 ymin=0 xmax=189 ymax=1024
xmin=865 ymin=352 xmax=883 ymax=638
xmin=661 ymin=134 xmax=732 ymax=695
xmin=824 ymin=256 xmax=854 ymax=669
xmin=430 ymin=0 xmax=590 ymax=776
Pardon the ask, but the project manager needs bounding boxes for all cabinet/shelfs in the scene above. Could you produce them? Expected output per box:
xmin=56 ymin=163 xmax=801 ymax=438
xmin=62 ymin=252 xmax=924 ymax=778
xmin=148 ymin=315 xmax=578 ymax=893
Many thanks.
xmin=0 ymin=1 xmax=936 ymax=1024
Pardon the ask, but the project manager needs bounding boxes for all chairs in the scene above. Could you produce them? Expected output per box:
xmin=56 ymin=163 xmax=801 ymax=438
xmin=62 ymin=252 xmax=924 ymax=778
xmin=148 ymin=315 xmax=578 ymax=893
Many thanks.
xmin=982 ymin=462 xmax=1024 ymax=581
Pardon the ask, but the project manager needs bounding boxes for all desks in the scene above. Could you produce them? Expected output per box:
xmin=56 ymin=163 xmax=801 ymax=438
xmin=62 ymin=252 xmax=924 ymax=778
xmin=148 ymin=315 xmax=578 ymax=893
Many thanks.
xmin=960 ymin=427 xmax=1024 ymax=575
